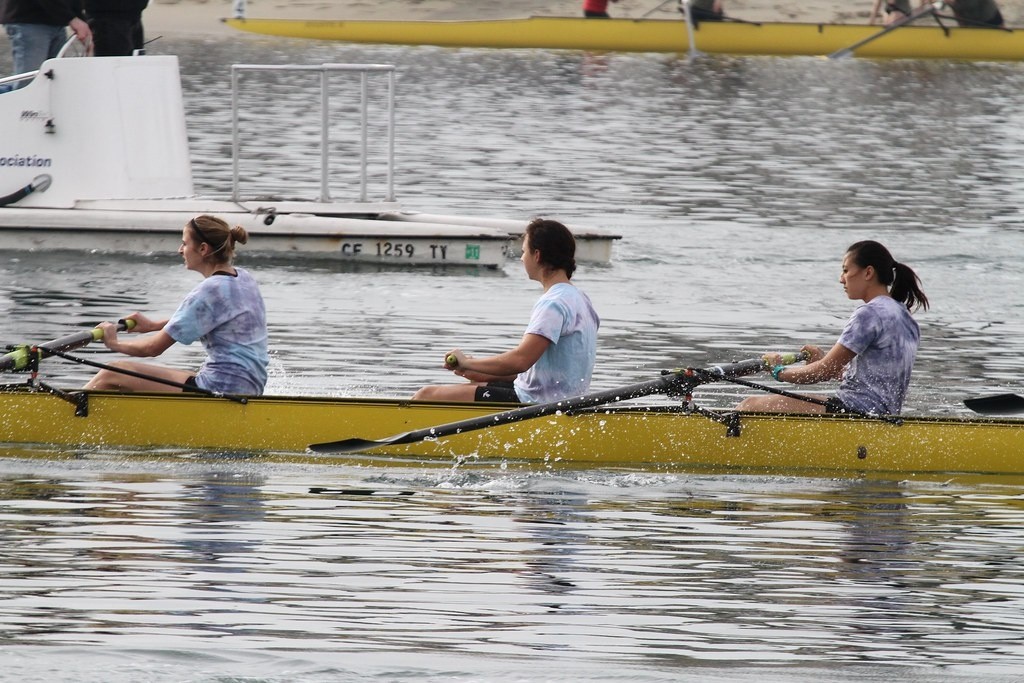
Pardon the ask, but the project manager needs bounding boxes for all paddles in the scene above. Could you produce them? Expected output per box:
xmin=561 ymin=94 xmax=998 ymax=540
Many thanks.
xmin=681 ymin=0 xmax=710 ymax=66
xmin=963 ymin=393 xmax=1024 ymax=414
xmin=0 ymin=318 xmax=137 ymax=373
xmin=305 ymin=348 xmax=814 ymax=457
xmin=445 ymin=354 xmax=459 ymax=368
xmin=825 ymin=1 xmax=946 ymax=61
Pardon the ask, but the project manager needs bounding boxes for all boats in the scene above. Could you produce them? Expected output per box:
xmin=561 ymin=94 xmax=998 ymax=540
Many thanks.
xmin=1 ymin=377 xmax=1024 ymax=476
xmin=224 ymin=15 xmax=1024 ymax=59
xmin=0 ymin=17 xmax=624 ymax=269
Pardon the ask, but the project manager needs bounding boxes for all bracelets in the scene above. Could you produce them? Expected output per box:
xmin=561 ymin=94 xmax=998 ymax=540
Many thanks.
xmin=772 ymin=365 xmax=786 ymax=381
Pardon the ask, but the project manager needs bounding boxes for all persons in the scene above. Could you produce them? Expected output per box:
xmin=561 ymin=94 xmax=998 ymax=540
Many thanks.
xmin=870 ymin=0 xmax=1005 ymax=29
xmin=0 ymin=0 xmax=148 ymax=90
xmin=735 ymin=240 xmax=930 ymax=415
xmin=583 ymin=0 xmax=618 ymax=17
xmin=680 ymin=0 xmax=723 ymax=21
xmin=81 ymin=215 xmax=269 ymax=397
xmin=410 ymin=218 xmax=599 ymax=403
